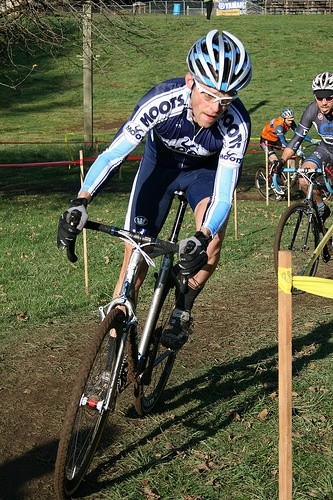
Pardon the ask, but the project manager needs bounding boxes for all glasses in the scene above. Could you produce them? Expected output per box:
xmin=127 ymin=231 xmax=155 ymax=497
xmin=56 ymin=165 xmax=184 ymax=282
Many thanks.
xmin=194 ymin=79 xmax=238 ymax=106
xmin=317 ymin=97 xmax=333 ymax=101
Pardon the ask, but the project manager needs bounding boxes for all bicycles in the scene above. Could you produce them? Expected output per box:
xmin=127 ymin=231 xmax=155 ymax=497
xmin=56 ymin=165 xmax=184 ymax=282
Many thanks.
xmin=255 ymin=139 xmax=333 ymax=295
xmin=54 ymin=191 xmax=196 ymax=500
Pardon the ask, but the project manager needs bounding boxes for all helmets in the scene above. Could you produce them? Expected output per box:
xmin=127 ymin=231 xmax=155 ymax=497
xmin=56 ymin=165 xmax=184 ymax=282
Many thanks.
xmin=280 ymin=109 xmax=294 ymax=119
xmin=312 ymin=72 xmax=333 ymax=91
xmin=186 ymin=30 xmax=253 ymax=93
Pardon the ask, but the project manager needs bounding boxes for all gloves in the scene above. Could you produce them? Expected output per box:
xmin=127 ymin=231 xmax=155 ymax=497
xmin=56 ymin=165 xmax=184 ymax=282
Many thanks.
xmin=311 ymin=139 xmax=322 ymax=144
xmin=297 ymin=149 xmax=304 ymax=157
xmin=179 ymin=230 xmax=212 ymax=276
xmin=56 ymin=197 xmax=89 ymax=251
xmin=269 ymin=158 xmax=286 ymax=175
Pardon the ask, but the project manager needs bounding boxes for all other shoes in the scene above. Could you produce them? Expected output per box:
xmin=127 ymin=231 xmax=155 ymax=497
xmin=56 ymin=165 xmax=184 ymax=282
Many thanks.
xmin=312 ymin=205 xmax=331 ymax=233
xmin=271 ymin=183 xmax=285 ymax=195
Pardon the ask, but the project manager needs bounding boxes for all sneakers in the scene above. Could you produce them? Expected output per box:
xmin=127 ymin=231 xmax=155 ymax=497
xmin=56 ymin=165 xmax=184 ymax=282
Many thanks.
xmin=87 ymin=371 xmax=127 ymax=410
xmin=159 ymin=309 xmax=193 ymax=350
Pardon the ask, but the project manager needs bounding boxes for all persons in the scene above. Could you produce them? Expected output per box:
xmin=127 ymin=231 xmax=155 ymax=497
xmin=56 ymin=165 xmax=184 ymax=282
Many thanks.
xmin=57 ymin=30 xmax=253 ymax=412
xmin=269 ymin=72 xmax=333 ymax=236
xmin=260 ymin=109 xmax=320 ymax=195
